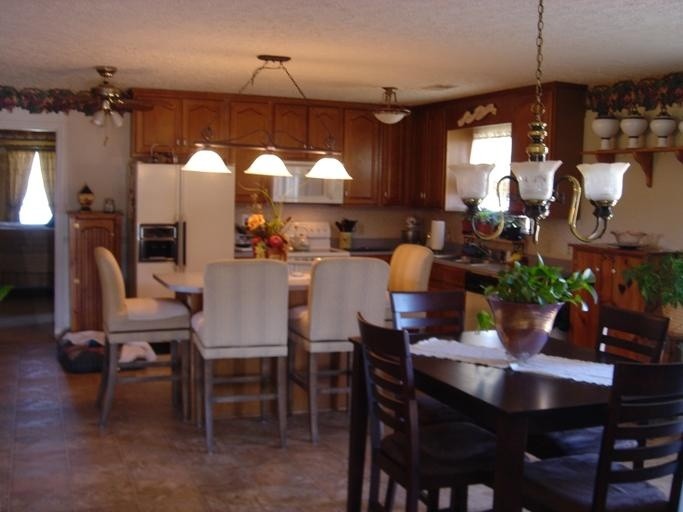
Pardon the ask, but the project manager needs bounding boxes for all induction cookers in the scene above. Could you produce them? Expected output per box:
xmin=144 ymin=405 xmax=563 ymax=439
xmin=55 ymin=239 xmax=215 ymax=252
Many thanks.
xmin=285 ymin=220 xmax=350 ymax=265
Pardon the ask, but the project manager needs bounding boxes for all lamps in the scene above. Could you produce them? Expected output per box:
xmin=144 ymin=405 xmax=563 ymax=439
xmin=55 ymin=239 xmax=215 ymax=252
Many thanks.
xmin=372 ymin=86 xmax=410 ymax=125
xmin=89 ymin=66 xmax=126 ymax=128
xmin=448 ymin=0 xmax=631 ymax=242
xmin=179 ymin=55 xmax=354 ymax=181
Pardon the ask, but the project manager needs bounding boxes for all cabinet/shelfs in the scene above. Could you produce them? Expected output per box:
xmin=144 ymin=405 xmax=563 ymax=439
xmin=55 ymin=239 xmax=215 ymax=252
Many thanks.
xmin=413 ymin=102 xmax=447 ymax=211
xmin=579 ymin=148 xmax=683 ymax=187
xmin=565 ymin=241 xmax=659 ymax=361
xmin=67 ymin=210 xmax=123 ymax=331
xmin=510 ymin=80 xmax=586 ymax=220
xmin=130 ymin=85 xmax=413 ymax=208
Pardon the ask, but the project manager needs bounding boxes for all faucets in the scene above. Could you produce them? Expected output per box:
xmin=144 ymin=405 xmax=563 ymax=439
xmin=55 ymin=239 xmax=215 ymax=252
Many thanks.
xmin=468 ymin=241 xmax=492 ymax=258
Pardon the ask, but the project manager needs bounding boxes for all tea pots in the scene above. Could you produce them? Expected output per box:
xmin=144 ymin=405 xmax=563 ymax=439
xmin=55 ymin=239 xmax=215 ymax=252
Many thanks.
xmin=75 ymin=182 xmax=94 ymax=213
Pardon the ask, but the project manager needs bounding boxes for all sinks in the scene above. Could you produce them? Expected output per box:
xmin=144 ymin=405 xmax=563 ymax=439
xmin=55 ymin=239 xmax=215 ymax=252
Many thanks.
xmin=438 ymin=256 xmax=488 ymax=265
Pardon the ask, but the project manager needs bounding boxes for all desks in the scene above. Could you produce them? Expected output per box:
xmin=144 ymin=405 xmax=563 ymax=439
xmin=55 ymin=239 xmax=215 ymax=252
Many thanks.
xmin=344 ymin=325 xmax=650 ymax=511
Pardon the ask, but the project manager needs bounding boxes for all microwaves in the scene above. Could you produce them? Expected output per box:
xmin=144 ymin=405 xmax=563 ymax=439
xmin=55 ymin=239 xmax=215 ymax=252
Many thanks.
xmin=271 ymin=160 xmax=344 ymax=206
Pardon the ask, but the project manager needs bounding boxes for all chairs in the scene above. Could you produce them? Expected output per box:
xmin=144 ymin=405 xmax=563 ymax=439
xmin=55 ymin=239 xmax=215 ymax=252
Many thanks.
xmin=189 ymin=259 xmax=289 ymax=449
xmin=389 ymin=291 xmax=467 ymax=428
xmin=355 ymin=310 xmax=501 ymax=511
xmin=505 ymin=362 xmax=681 ymax=512
xmin=288 ymin=257 xmax=390 ymax=444
xmin=526 ymin=305 xmax=671 ymax=479
xmin=94 ymin=246 xmax=192 ymax=426
xmin=387 ymin=243 xmax=435 ymax=334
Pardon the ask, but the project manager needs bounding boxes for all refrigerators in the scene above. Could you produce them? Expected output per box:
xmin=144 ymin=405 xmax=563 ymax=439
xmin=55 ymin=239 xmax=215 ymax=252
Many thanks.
xmin=129 ymin=159 xmax=236 ymax=299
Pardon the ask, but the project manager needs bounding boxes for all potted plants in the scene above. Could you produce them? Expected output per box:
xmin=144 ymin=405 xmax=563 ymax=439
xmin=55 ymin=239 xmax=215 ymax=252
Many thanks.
xmin=476 ymin=260 xmax=600 ymax=360
xmin=620 ymin=247 xmax=683 ymax=339
